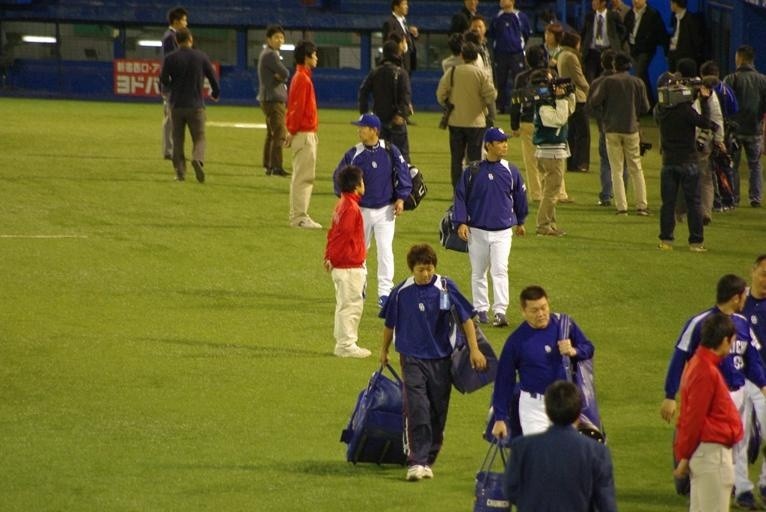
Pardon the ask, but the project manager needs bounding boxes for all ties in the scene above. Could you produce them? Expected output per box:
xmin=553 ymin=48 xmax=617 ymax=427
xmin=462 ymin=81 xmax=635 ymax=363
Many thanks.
xmin=595 ymin=15 xmax=602 ymax=49
xmin=403 ymin=21 xmax=411 ymax=50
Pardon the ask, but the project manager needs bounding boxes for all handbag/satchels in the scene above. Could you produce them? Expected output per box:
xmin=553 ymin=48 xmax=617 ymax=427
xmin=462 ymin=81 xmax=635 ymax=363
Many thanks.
xmin=450 ymin=320 xmax=498 ymax=395
xmin=439 ymin=102 xmax=456 ymax=129
xmin=340 ymin=364 xmax=406 ymax=465
xmin=474 ymin=470 xmax=511 ymax=511
xmin=574 ymin=355 xmax=606 ymax=443
xmin=439 ymin=205 xmax=469 ymax=252
xmin=391 ymin=163 xmax=427 ymax=211
xmin=483 ymin=384 xmax=522 ymax=448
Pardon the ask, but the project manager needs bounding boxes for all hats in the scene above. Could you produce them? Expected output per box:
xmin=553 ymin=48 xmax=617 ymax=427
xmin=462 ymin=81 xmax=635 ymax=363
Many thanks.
xmin=350 ymin=112 xmax=381 ymax=130
xmin=484 ymin=127 xmax=512 ymax=143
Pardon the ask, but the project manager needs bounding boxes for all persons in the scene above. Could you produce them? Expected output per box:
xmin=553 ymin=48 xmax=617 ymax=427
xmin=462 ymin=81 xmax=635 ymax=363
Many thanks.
xmin=254 ymin=25 xmax=293 ymax=178
xmin=377 ymin=242 xmax=492 ymax=484
xmin=731 ymin=253 xmax=766 ymax=510
xmin=660 ymin=273 xmax=765 ymax=510
xmin=334 ymin=113 xmax=413 ymax=308
xmin=361 ymin=1 xmax=418 ymax=163
xmin=157 ymin=8 xmax=193 ymax=162
xmin=438 ymin=1 xmax=766 ymax=251
xmin=158 ymin=27 xmax=222 ymax=182
xmin=492 ymin=285 xmax=608 ymax=446
xmin=319 ymin=163 xmax=374 ymax=361
xmin=502 ymin=380 xmax=619 ymax=512
xmin=282 ymin=39 xmax=324 ymax=229
xmin=675 ymin=313 xmax=745 ymax=511
xmin=451 ymin=126 xmax=529 ymax=327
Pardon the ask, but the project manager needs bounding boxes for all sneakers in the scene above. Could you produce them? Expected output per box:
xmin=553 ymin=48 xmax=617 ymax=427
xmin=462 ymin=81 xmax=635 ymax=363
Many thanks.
xmin=536 ymin=229 xmax=561 ymax=237
xmin=599 ymin=198 xmax=612 ymax=206
xmin=406 ymin=465 xmax=424 ymax=480
xmin=294 ymin=216 xmax=323 ymax=228
xmin=689 ymin=246 xmax=707 ymax=252
xmin=736 ymin=491 xmax=757 ymax=510
xmin=636 ymin=207 xmax=653 ymax=216
xmin=494 ymin=313 xmax=507 ymax=327
xmin=271 ymin=167 xmax=291 ymax=176
xmin=478 ymin=311 xmax=489 ymax=323
xmin=423 ymin=465 xmax=432 ymax=478
xmin=559 ymin=197 xmax=573 ymax=203
xmin=266 ymin=168 xmax=271 ymax=175
xmin=616 ymin=210 xmax=627 ymax=216
xmin=378 ymin=296 xmax=388 ymax=307
xmin=336 ymin=346 xmax=370 ymax=359
xmin=165 ymin=153 xmax=173 ymax=160
xmin=554 ymin=229 xmax=566 ymax=235
xmin=657 ymin=243 xmax=672 ymax=250
xmin=191 ymin=159 xmax=204 ymax=182
